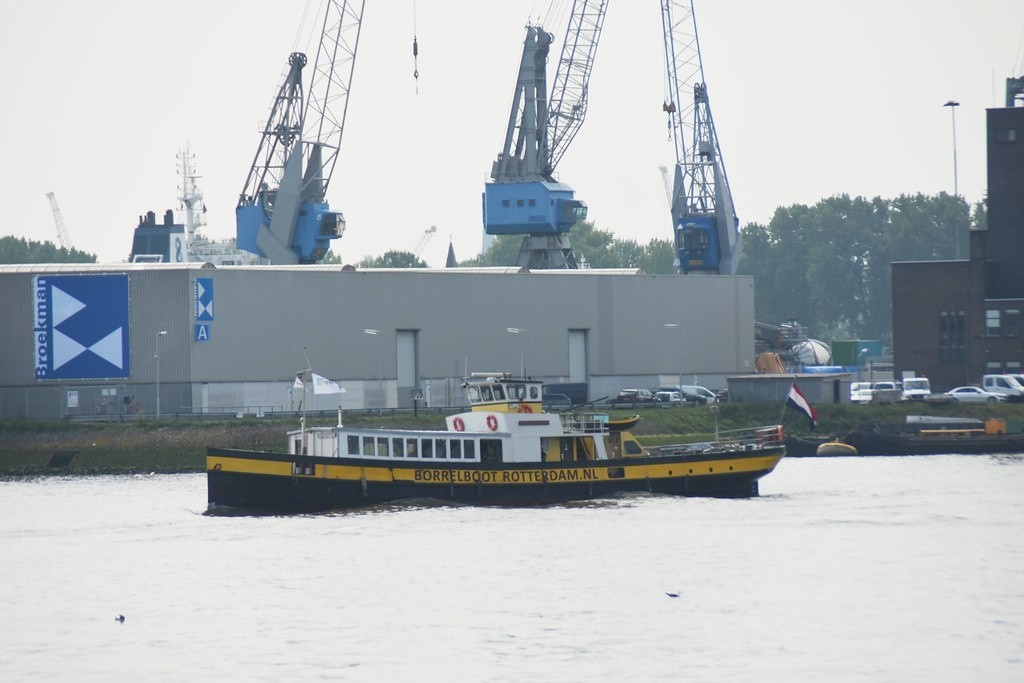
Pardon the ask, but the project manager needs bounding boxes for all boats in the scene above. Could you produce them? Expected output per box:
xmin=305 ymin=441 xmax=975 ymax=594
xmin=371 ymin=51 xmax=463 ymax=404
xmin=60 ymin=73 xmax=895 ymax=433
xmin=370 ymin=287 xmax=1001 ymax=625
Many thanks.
xmin=205 ymin=367 xmax=786 ymax=517
xmin=784 ymin=414 xmax=1024 ymax=457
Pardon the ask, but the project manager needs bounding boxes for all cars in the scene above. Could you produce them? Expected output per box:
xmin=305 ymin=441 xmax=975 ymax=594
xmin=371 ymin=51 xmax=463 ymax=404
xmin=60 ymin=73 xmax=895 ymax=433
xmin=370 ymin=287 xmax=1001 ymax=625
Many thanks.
xmin=902 ymin=378 xmax=931 ymax=401
xmin=850 ymin=378 xmax=902 ymax=403
xmin=532 ymin=393 xmax=571 ymax=405
xmin=618 ymin=387 xmax=708 ymax=405
xmin=715 ymin=388 xmax=728 ymax=403
xmin=942 ymin=374 xmax=1024 ymax=403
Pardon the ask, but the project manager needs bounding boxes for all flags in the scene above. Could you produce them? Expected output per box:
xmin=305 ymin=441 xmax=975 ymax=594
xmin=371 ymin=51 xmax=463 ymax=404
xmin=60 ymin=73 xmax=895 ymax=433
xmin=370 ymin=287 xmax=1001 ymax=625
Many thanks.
xmin=294 ymin=377 xmax=309 ymax=392
xmin=311 ymin=373 xmax=346 ymax=394
xmin=785 ymin=382 xmax=817 ymax=431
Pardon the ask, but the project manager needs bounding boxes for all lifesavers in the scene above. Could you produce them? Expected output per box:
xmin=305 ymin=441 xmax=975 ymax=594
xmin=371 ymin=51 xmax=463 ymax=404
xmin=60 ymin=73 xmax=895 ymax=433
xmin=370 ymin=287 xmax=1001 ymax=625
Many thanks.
xmin=518 ymin=405 xmax=534 ymax=413
xmin=486 ymin=415 xmax=497 ymax=431
xmin=453 ymin=417 xmax=464 ymax=431
xmin=777 ymin=427 xmax=783 ymax=440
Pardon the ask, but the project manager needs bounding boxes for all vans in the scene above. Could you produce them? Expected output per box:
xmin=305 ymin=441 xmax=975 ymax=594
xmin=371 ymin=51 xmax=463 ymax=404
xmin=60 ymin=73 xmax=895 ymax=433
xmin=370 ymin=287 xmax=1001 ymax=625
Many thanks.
xmin=676 ymin=385 xmax=719 ymax=404
xmin=533 ymin=383 xmax=588 ymax=404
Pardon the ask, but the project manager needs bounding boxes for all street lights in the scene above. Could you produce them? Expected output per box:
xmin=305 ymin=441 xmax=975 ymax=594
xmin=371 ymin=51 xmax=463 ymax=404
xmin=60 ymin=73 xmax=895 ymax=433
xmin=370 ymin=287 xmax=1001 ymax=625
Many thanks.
xmin=508 ymin=328 xmax=528 ymax=381
xmin=943 ymin=100 xmax=960 ymax=197
xmin=365 ymin=329 xmax=383 ymax=417
xmin=156 ymin=331 xmax=167 ymax=417
xmin=857 ymin=348 xmax=868 ymax=389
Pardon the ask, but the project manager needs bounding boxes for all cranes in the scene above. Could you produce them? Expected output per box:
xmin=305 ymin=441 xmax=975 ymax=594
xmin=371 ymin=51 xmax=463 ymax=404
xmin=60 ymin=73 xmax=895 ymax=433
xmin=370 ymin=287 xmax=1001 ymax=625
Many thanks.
xmin=236 ymin=0 xmax=438 ymax=266
xmin=660 ymin=0 xmax=742 ymax=274
xmin=47 ymin=192 xmax=69 ymax=247
xmin=482 ymin=0 xmax=610 ymax=270
